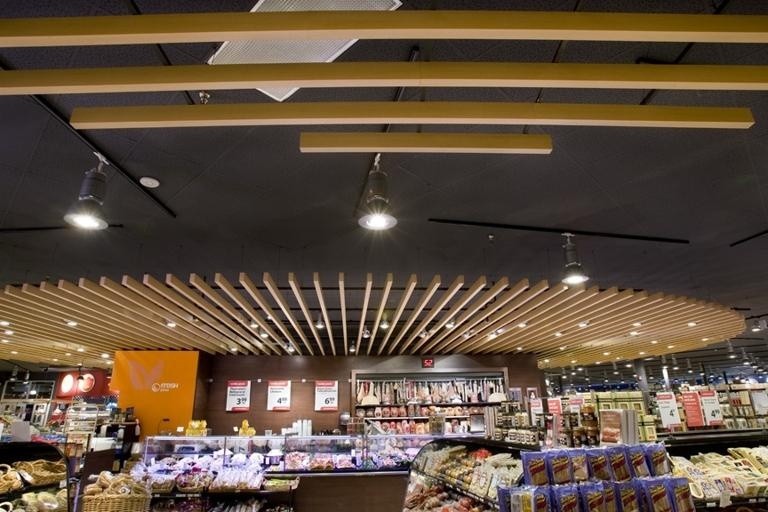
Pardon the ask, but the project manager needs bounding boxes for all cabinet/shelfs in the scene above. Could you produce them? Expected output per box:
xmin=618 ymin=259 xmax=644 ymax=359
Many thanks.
xmin=663 ymin=428 xmax=768 ymax=512
xmin=349 ymin=365 xmax=507 ymax=434
xmin=142 ymin=431 xmax=467 ymax=476
xmin=400 ymin=436 xmax=539 ymax=511
xmin=0 ymin=441 xmax=76 ymax=512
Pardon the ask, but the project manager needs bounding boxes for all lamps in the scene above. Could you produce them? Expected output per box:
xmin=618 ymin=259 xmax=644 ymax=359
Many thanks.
xmin=57 ymin=146 xmax=115 ymax=232
xmin=356 ymin=151 xmax=403 ymax=234
xmin=556 ymin=234 xmax=594 ymax=284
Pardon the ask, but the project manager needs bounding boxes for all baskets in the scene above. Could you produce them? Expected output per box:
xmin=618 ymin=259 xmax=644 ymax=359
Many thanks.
xmin=152 ymin=478 xmax=175 ymax=494
xmin=206 ymin=474 xmax=264 ymax=494
xmin=13 ymin=459 xmax=68 ymax=486
xmin=79 ymin=493 xmax=153 ymax=512
xmin=176 ymin=484 xmax=203 ymax=494
xmin=264 ymin=479 xmax=296 ymax=493
